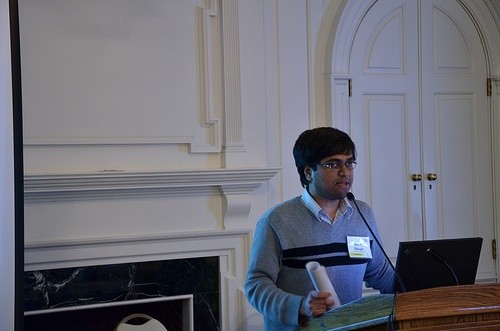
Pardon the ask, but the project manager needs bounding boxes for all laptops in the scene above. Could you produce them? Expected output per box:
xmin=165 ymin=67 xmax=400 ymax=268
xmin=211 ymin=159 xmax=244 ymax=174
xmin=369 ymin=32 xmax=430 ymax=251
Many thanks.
xmin=393 ymin=237 xmax=483 ymax=293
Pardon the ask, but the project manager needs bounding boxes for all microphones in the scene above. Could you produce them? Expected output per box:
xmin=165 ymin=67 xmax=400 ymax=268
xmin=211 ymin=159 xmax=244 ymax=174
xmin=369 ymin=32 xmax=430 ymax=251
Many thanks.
xmin=427 ymin=248 xmax=460 ymax=287
xmin=346 ymin=191 xmax=407 ymax=293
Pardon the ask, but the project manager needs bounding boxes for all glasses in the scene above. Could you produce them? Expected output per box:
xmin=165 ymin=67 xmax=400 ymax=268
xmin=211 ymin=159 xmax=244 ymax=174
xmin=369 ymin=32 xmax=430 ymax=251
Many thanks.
xmin=317 ymin=161 xmax=357 ymax=170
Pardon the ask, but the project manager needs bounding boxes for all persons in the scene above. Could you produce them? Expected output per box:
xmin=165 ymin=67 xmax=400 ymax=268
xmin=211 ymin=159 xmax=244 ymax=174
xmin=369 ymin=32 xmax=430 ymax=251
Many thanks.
xmin=242 ymin=124 xmax=395 ymax=331
